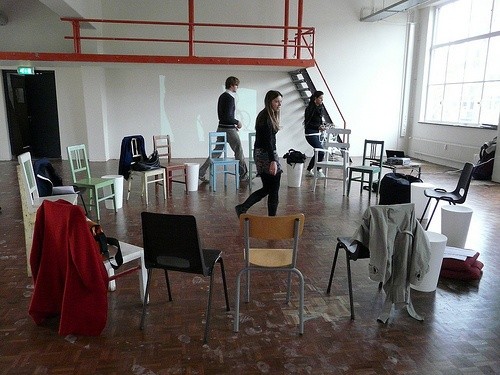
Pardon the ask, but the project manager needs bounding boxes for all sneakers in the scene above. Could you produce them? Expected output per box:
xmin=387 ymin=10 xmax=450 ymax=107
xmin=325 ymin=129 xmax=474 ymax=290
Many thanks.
xmin=305 ymin=168 xmax=315 ymax=176
xmin=317 ymin=169 xmax=325 ymax=177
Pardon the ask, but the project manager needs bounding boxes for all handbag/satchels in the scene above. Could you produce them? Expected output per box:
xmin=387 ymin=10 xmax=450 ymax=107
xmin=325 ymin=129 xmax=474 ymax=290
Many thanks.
xmin=132 ymin=149 xmax=160 ymax=171
xmin=283 ymin=149 xmax=306 ymax=164
xmin=363 ymin=180 xmax=382 ymax=192
xmin=85 ymin=220 xmax=123 ymax=270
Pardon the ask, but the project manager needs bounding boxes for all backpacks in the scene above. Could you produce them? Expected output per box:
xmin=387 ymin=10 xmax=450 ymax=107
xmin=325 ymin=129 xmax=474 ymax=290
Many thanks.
xmin=472 ymin=144 xmax=495 ymax=181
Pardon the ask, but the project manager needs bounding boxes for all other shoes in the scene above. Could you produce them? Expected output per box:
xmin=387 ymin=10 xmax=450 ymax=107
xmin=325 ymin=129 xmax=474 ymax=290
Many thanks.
xmin=241 ymin=173 xmax=256 ymax=182
xmin=235 ymin=204 xmax=246 ymax=218
xmin=199 ymin=175 xmax=209 ymax=183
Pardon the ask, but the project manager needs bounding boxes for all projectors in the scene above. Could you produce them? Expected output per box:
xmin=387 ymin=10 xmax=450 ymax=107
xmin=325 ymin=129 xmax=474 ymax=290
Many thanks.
xmin=387 ymin=156 xmax=410 ymax=166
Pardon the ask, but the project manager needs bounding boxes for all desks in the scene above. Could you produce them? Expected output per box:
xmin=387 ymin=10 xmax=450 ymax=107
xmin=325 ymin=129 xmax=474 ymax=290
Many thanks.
xmin=101 ymin=175 xmax=124 ymax=209
xmin=442 ymin=206 xmax=473 ymax=250
xmin=410 ymin=230 xmax=447 ymax=292
xmin=369 ymin=162 xmax=421 ymax=179
xmin=286 ymin=163 xmax=304 ymax=187
xmin=184 ymin=162 xmax=200 ymax=191
xmin=411 ymin=182 xmax=435 ymax=219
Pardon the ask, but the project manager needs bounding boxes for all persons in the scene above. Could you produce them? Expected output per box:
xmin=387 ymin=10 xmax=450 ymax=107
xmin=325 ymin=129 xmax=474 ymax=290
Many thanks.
xmin=199 ymin=76 xmax=255 ymax=182
xmin=305 ymin=91 xmax=325 ymax=177
xmin=235 ymin=90 xmax=282 ymax=219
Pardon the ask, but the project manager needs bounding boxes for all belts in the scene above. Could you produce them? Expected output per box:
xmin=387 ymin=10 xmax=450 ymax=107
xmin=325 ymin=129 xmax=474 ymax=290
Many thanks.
xmin=219 ymin=125 xmax=235 ymax=128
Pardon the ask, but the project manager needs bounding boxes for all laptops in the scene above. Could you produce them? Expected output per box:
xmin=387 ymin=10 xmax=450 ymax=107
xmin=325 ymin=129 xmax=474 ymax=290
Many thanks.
xmin=385 ymin=149 xmax=404 ymax=159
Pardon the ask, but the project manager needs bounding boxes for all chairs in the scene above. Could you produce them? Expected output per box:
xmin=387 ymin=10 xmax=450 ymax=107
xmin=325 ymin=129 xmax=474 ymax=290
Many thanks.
xmin=66 ymin=144 xmax=118 ymax=220
xmin=140 ymin=212 xmax=230 ymax=343
xmin=420 ymin=162 xmax=475 ymax=231
xmin=154 ymin=135 xmax=189 ymax=197
xmin=210 ymin=132 xmax=239 ymax=192
xmin=347 ymin=139 xmax=384 ymax=200
xmin=314 ymin=130 xmax=351 ymax=195
xmin=15 ymin=152 xmax=88 ymax=277
xmin=326 ymin=204 xmax=430 ymax=325
xmin=248 ymin=133 xmax=256 ymax=190
xmin=119 ymin=135 xmax=167 ymax=204
xmin=28 ymin=199 xmax=150 ymax=339
xmin=234 ymin=214 xmax=305 ymax=336
